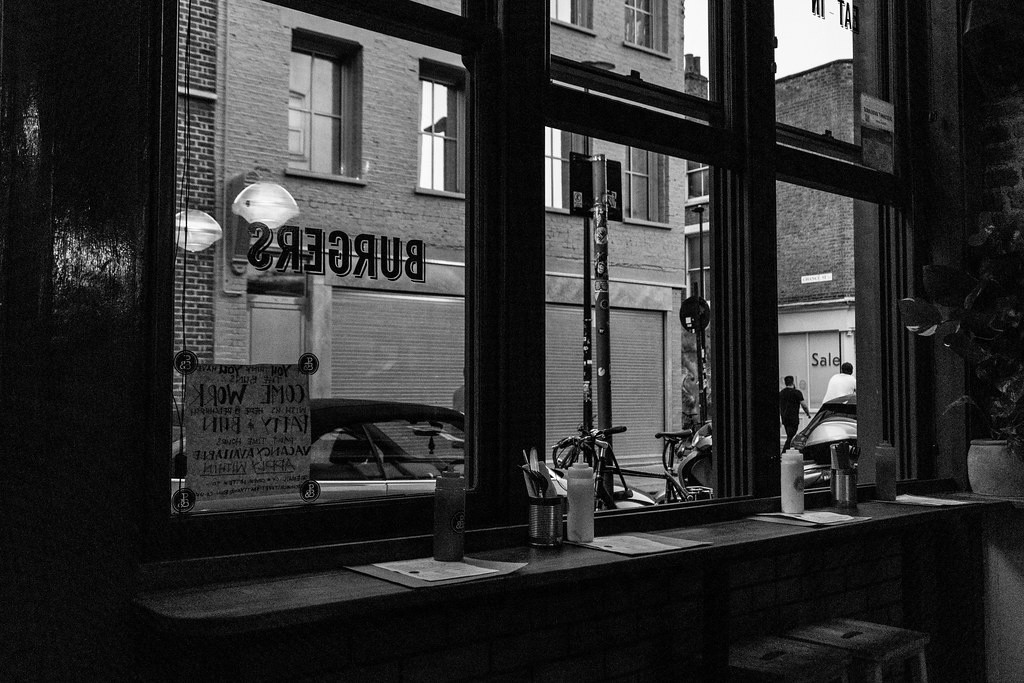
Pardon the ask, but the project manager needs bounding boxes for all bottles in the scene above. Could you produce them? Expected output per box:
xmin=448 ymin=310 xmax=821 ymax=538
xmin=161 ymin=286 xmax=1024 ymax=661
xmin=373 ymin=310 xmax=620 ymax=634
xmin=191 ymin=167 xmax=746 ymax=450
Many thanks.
xmin=780 ymin=444 xmax=805 ymax=514
xmin=875 ymin=440 xmax=897 ymax=500
xmin=433 ymin=466 xmax=465 ymax=561
xmin=565 ymin=450 xmax=595 ymax=543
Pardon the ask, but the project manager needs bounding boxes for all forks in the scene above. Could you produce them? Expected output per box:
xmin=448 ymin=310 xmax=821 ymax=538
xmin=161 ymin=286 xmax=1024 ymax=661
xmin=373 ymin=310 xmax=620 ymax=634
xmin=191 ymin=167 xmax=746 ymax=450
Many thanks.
xmin=850 ymin=445 xmax=862 ymax=468
xmin=516 ymin=464 xmax=548 ymax=499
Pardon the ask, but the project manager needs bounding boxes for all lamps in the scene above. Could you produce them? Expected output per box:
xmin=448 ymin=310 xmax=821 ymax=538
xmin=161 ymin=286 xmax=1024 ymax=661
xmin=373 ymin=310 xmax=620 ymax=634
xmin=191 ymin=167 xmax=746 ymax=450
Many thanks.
xmin=176 ymin=208 xmax=225 ymax=255
xmin=231 ymin=179 xmax=301 ymax=228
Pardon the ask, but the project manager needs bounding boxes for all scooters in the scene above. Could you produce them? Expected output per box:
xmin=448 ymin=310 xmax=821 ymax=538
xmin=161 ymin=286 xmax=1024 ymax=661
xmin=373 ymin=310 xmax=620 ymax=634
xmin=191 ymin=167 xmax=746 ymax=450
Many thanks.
xmin=657 ymin=415 xmax=857 ymax=502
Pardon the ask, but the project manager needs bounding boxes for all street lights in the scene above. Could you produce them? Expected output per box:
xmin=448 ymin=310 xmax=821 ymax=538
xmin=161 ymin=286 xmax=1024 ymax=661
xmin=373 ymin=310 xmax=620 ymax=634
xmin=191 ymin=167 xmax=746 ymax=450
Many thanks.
xmin=691 ymin=205 xmax=708 ymax=421
xmin=580 ymin=59 xmax=616 ymax=432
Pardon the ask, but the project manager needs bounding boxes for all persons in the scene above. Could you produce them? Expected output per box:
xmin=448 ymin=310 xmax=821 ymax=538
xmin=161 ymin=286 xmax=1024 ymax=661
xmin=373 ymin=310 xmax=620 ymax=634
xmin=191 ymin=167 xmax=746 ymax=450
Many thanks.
xmin=822 ymin=362 xmax=856 ymax=404
xmin=779 ymin=376 xmax=811 ymax=453
xmin=451 ymin=367 xmax=465 ymax=450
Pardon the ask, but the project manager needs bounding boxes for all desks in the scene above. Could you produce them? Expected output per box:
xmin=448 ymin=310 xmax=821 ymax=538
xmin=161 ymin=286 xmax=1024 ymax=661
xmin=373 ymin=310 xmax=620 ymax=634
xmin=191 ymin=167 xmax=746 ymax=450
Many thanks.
xmin=126 ymin=491 xmax=1015 ymax=646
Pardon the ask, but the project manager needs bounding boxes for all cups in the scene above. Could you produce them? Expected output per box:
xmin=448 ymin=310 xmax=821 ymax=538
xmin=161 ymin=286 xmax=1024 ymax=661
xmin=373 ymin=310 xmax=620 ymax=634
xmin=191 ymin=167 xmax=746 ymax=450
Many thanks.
xmin=527 ymin=496 xmax=565 ymax=549
xmin=829 ymin=469 xmax=858 ymax=509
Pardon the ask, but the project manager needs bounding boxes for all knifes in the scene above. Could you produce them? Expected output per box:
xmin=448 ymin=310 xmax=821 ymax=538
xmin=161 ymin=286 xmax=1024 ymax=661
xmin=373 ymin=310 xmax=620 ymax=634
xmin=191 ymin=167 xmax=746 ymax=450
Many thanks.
xmin=530 ymin=446 xmax=539 ymax=473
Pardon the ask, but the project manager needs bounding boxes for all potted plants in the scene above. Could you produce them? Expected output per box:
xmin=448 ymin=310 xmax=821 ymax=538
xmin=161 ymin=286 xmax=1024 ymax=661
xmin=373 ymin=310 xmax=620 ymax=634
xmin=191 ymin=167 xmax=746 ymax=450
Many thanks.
xmin=897 ymin=213 xmax=1023 ymax=501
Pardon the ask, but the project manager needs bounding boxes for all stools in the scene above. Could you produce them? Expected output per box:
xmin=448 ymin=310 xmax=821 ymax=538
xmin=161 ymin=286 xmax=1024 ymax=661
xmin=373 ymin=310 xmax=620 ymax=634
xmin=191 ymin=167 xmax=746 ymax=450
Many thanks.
xmin=694 ymin=614 xmax=931 ymax=683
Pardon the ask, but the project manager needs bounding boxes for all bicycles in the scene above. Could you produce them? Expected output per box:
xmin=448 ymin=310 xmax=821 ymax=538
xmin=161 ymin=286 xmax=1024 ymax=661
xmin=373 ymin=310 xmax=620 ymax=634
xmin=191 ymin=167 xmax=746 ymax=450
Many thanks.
xmin=551 ymin=426 xmax=714 ymax=503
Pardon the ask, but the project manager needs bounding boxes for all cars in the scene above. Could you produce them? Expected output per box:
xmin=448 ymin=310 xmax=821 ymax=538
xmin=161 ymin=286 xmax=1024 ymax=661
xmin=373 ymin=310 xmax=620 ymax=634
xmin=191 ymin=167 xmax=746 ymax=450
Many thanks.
xmin=171 ymin=400 xmax=657 ymax=512
